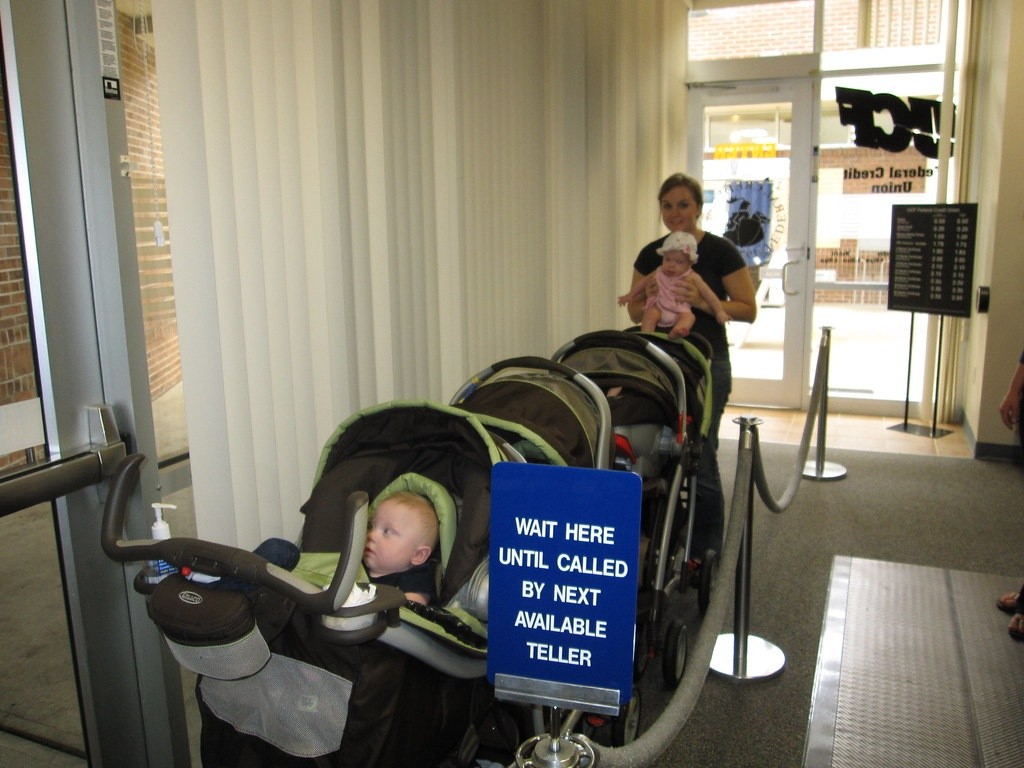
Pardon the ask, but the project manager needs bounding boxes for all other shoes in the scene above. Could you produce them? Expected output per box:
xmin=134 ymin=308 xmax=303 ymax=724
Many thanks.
xmin=996 ymin=592 xmax=1024 ymax=636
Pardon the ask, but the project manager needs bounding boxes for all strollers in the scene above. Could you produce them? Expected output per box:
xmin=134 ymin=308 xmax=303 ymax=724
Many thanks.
xmin=101 ymin=324 xmax=718 ymax=768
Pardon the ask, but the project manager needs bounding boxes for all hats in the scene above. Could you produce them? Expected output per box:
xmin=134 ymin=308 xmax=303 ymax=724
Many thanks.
xmin=656 ymin=231 xmax=698 ymax=263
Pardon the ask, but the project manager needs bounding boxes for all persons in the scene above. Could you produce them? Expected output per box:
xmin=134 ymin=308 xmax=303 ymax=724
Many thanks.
xmin=338 ymin=491 xmax=438 ymax=699
xmin=996 ymin=350 xmax=1024 ymax=641
xmin=617 ymin=172 xmax=756 ymax=568
